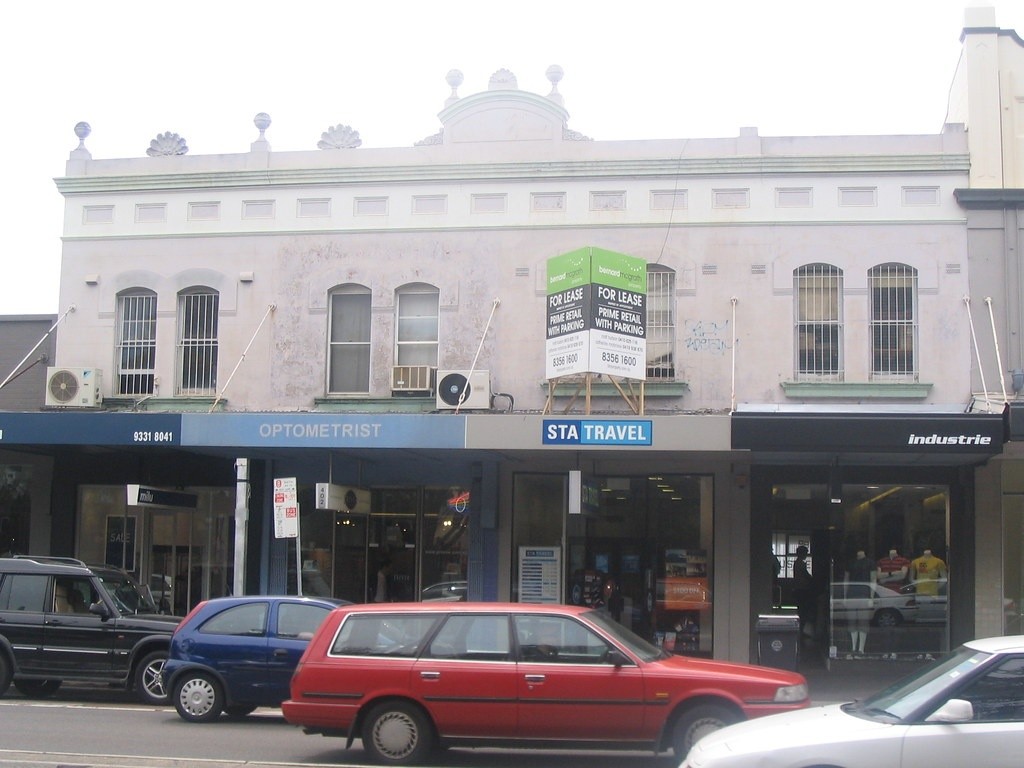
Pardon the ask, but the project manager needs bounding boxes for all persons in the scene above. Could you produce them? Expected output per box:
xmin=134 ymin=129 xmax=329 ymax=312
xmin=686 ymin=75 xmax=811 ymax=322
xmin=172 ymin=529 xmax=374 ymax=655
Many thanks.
xmin=531 ymin=623 xmax=559 ymax=662
xmin=843 ymin=551 xmax=876 ymax=654
xmin=876 ymin=549 xmax=910 ymax=659
xmin=794 ymin=546 xmax=812 ymax=639
xmin=910 ymin=550 xmax=947 ymax=660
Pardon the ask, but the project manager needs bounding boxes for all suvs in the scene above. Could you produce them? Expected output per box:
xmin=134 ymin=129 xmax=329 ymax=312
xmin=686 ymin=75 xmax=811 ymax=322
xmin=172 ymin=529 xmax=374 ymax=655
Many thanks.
xmin=0 ymin=555 xmax=186 ymax=705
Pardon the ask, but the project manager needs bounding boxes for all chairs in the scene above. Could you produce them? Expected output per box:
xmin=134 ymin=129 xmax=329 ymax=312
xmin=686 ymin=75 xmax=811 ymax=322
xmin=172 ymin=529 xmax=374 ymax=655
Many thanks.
xmin=53 ymin=585 xmax=74 ymax=614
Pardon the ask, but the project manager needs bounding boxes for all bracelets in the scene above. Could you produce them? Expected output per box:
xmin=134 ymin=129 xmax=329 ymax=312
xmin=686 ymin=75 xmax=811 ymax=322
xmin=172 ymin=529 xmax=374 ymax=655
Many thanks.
xmin=889 ymin=572 xmax=891 ymax=576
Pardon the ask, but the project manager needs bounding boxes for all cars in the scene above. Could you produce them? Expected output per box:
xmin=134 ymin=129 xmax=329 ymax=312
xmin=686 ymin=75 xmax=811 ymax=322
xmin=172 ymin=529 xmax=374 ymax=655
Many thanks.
xmin=161 ymin=594 xmax=356 ymax=725
xmin=283 ymin=602 xmax=813 ymax=765
xmin=422 ymin=581 xmax=467 ymax=602
xmin=829 ymin=581 xmax=919 ymax=626
xmin=678 ymin=635 xmax=1024 ymax=768
xmin=898 ymin=579 xmax=1017 ymax=625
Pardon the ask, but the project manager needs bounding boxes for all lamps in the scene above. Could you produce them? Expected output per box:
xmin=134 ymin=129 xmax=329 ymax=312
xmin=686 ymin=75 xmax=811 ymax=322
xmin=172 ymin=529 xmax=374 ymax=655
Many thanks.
xmin=240 ymin=271 xmax=255 ymax=283
xmin=85 ymin=274 xmax=99 ymax=285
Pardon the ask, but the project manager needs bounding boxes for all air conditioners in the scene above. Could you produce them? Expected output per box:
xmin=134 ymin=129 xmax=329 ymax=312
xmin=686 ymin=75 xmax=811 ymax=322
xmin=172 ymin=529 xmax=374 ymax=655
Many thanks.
xmin=390 ymin=366 xmax=436 ymax=392
xmin=45 ymin=366 xmax=103 ymax=408
xmin=436 ymin=370 xmax=492 ymax=409
